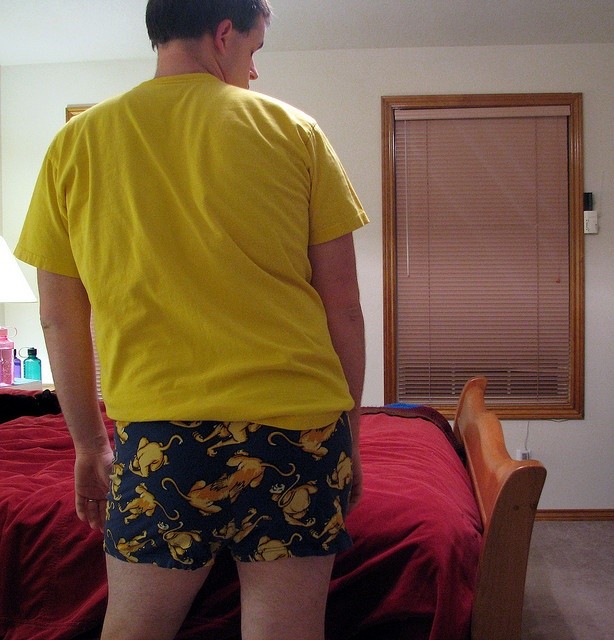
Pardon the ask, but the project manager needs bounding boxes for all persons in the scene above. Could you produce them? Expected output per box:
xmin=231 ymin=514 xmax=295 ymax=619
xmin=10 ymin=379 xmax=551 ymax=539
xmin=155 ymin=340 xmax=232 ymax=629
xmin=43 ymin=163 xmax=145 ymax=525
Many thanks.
xmin=36 ymin=0 xmax=365 ymax=640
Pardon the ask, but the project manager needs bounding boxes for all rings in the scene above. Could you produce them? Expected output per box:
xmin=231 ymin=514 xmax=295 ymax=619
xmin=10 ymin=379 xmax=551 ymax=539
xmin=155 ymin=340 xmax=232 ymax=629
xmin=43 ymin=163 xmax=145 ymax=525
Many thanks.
xmin=87 ymin=499 xmax=97 ymax=503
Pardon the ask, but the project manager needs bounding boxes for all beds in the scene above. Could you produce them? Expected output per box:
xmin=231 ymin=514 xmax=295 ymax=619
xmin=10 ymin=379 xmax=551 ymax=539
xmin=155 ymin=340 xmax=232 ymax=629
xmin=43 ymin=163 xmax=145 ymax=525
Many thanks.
xmin=0 ymin=377 xmax=547 ymax=639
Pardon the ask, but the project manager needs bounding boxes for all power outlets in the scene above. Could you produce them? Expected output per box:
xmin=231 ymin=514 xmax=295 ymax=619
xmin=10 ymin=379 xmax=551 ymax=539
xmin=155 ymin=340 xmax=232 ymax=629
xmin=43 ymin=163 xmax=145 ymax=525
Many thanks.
xmin=517 ymin=450 xmax=531 ymax=460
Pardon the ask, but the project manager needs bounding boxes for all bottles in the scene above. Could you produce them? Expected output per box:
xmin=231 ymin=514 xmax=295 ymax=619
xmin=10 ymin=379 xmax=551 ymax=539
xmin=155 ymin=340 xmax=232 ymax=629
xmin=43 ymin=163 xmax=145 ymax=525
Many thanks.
xmin=13 ymin=348 xmax=22 ymax=379
xmin=23 ymin=347 xmax=42 ymax=381
xmin=0 ymin=326 xmax=18 ymax=387
